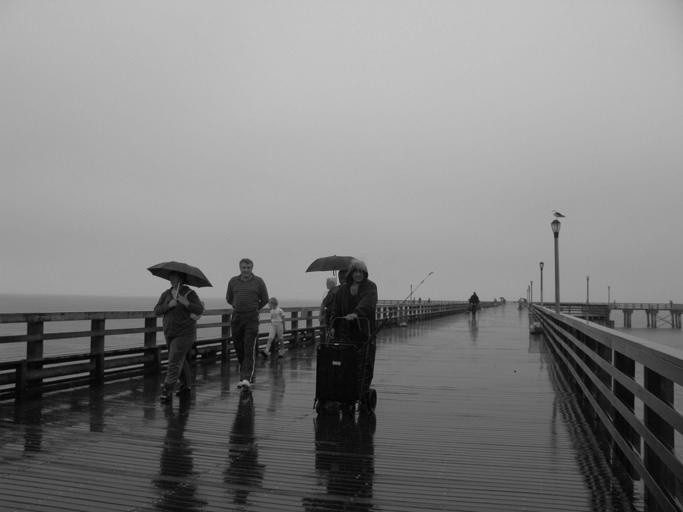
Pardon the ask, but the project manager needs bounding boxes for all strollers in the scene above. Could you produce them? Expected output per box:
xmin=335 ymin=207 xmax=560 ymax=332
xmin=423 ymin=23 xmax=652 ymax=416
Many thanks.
xmin=305 ymin=312 xmax=380 ymax=419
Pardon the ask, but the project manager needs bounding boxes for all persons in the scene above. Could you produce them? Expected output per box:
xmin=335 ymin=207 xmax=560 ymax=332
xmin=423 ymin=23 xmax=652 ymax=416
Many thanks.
xmin=323 ymin=269 xmax=349 ymax=343
xmin=467 ymin=292 xmax=480 ymax=313
xmin=318 ymin=277 xmax=337 ymax=345
xmin=225 ymin=258 xmax=269 ymax=388
xmin=260 ymin=297 xmax=286 ymax=358
xmin=327 ymin=260 xmax=378 ymax=414
xmin=154 ymin=273 xmax=203 ymax=402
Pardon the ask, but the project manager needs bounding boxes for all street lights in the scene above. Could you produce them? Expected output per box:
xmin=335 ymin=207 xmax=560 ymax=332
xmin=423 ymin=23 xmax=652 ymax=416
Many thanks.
xmin=523 ymin=219 xmax=612 ymax=307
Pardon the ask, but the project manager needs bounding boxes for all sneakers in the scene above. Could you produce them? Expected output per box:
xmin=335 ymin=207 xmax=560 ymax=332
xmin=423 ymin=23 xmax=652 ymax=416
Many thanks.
xmin=260 ymin=350 xmax=268 ymax=358
xmin=176 ymin=385 xmax=191 ymax=395
xmin=236 ymin=381 xmax=241 ymax=388
xmin=278 ymin=352 xmax=283 ymax=358
xmin=159 ymin=382 xmax=173 ymax=400
xmin=242 ymin=379 xmax=248 ymax=387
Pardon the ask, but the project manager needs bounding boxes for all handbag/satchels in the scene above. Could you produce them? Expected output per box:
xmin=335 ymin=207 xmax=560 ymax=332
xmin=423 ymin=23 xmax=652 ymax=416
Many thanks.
xmin=184 ymin=289 xmax=206 ymax=322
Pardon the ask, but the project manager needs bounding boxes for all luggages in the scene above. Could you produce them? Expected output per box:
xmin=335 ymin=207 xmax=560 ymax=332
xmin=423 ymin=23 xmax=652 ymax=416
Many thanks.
xmin=316 ymin=342 xmax=360 ymax=403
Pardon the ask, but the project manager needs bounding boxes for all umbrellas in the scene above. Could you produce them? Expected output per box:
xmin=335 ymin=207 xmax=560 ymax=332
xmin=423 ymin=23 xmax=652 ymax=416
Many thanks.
xmin=146 ymin=260 xmax=212 ymax=288
xmin=304 ymin=254 xmax=357 ymax=272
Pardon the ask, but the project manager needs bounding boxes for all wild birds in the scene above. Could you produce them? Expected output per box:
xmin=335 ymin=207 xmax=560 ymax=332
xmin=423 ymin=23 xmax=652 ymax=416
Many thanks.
xmin=552 ymin=209 xmax=566 ymax=220
